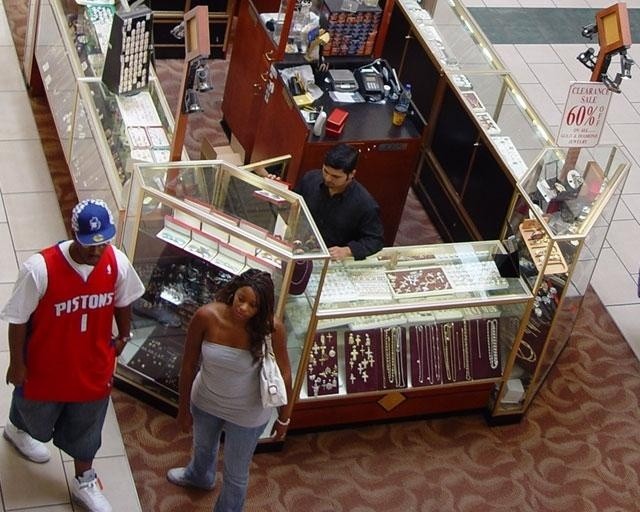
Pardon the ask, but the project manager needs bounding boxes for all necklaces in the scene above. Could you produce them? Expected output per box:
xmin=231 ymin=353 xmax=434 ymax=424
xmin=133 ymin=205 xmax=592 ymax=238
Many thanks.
xmin=502 ymin=307 xmax=542 ymax=363
xmin=382 ymin=319 xmax=499 ymax=388
xmin=457 ymin=291 xmax=498 ymax=316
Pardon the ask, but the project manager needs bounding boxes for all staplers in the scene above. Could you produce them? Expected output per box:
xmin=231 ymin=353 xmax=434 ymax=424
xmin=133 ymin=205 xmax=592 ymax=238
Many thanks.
xmin=288 ymin=76 xmax=301 ymax=96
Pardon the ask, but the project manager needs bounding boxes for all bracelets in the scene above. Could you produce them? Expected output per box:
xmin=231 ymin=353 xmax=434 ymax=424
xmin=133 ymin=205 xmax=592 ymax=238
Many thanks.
xmin=275 ymin=417 xmax=291 ymax=426
xmin=118 ymin=332 xmax=133 ymax=343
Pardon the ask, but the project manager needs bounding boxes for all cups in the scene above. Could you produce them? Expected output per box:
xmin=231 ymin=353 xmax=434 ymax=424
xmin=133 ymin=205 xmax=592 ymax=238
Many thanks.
xmin=393 ymin=104 xmax=408 ymax=127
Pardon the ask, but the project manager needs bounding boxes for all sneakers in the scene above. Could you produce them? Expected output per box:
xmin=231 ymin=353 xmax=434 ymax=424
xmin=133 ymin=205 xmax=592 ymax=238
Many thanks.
xmin=167 ymin=467 xmax=215 ymax=491
xmin=4 ymin=420 xmax=50 ymax=463
xmin=73 ymin=469 xmax=112 ymax=512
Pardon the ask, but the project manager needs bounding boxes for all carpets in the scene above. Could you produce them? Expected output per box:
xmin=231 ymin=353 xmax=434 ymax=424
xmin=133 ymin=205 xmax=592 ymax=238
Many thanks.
xmin=2 ymin=0 xmax=639 ymax=510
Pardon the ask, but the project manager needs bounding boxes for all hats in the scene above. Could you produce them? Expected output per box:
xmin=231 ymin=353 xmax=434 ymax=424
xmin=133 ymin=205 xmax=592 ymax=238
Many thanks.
xmin=71 ymin=199 xmax=116 ymax=246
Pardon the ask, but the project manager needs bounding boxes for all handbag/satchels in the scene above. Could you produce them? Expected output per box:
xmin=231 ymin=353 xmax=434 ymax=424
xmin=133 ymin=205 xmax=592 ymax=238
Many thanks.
xmin=259 ymin=335 xmax=286 ymax=408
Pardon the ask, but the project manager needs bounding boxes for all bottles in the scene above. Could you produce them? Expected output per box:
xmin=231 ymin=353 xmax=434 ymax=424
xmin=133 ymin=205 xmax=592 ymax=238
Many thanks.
xmin=398 ymin=85 xmax=413 ymax=109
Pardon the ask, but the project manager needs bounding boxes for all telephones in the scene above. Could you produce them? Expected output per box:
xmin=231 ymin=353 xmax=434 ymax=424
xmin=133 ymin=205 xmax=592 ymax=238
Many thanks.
xmin=356 ymin=69 xmax=383 ymax=93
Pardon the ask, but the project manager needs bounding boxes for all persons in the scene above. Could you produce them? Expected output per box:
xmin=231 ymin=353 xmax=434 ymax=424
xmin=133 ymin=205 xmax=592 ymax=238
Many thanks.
xmin=3 ymin=199 xmax=146 ymax=512
xmin=165 ymin=269 xmax=292 ymax=512
xmin=267 ymin=143 xmax=383 ymax=261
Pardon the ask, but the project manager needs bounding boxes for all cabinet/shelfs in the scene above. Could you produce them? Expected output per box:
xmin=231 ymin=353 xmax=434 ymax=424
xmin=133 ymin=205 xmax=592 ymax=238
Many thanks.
xmin=106 ymin=160 xmax=333 ymax=448
xmin=485 ymin=143 xmax=633 ymax=428
xmin=379 ymin=0 xmax=569 ymax=244
xmin=222 ymin=1 xmax=382 ymax=162
xmin=250 ymin=57 xmax=429 ymax=245
xmin=280 ymin=239 xmax=534 ymax=433
xmin=24 ymin=0 xmax=204 ymax=257
